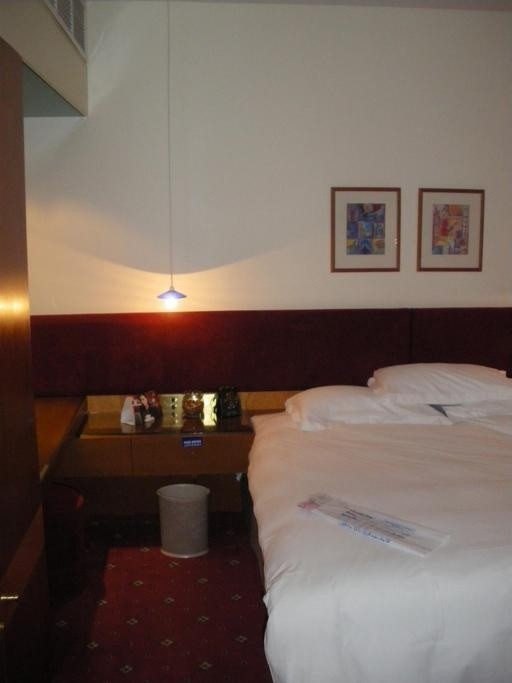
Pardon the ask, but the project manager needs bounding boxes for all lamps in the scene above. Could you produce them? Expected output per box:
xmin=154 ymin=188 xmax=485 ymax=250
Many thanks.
xmin=157 ymin=0 xmax=187 ymax=311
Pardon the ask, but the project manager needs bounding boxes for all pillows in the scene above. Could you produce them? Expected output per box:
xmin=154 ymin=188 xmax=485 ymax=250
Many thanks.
xmin=285 ymin=363 xmax=512 ymax=433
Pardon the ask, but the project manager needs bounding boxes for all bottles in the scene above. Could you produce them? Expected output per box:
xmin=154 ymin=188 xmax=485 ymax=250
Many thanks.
xmin=181 ymin=390 xmax=203 ymax=415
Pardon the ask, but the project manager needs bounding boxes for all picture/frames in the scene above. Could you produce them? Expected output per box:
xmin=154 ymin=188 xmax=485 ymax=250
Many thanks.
xmin=417 ymin=188 xmax=485 ymax=272
xmin=330 ymin=187 xmax=401 ymax=273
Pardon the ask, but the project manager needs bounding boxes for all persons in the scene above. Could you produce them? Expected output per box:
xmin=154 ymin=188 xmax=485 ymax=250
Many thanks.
xmin=138 ymin=392 xmax=162 ymax=430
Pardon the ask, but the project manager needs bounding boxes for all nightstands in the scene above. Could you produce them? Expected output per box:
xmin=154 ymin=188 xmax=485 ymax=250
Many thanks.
xmin=73 ymin=410 xmax=255 ymax=479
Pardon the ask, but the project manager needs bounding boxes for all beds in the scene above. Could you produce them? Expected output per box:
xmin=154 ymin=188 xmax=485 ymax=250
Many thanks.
xmin=246 ymin=413 xmax=512 ymax=683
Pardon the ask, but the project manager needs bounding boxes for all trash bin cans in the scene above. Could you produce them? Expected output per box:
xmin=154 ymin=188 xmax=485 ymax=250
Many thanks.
xmin=154 ymin=482 xmax=212 ymax=560
